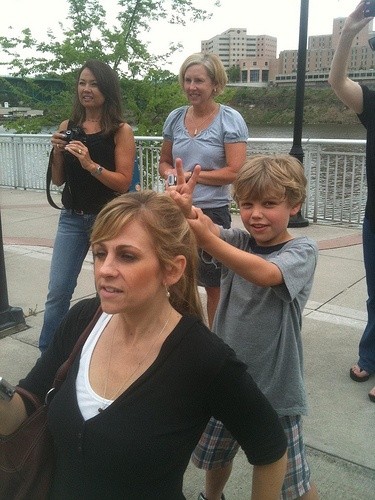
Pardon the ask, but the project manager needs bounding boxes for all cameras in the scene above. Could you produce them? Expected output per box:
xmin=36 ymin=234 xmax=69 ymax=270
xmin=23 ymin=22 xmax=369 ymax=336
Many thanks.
xmin=168 ymin=175 xmax=177 ymax=186
xmin=58 ymin=126 xmax=90 ymax=148
xmin=0 ymin=376 xmax=16 ymax=401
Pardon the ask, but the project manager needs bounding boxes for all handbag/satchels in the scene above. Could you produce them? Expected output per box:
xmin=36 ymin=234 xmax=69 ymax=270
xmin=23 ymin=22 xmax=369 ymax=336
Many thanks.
xmin=0 ymin=403 xmax=51 ymax=500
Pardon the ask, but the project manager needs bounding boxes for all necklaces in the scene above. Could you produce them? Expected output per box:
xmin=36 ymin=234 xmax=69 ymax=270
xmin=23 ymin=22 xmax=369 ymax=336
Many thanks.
xmin=192 ymin=108 xmax=217 ymax=136
xmin=87 ymin=120 xmax=100 ymax=122
xmin=98 ymin=310 xmax=173 ymax=413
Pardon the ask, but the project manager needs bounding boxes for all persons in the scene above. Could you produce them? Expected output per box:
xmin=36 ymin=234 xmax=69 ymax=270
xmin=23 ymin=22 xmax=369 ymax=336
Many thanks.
xmin=159 ymin=53 xmax=248 ymax=330
xmin=328 ymin=0 xmax=375 ymax=402
xmin=0 ymin=192 xmax=288 ymax=500
xmin=39 ymin=59 xmax=135 ymax=354
xmin=165 ymin=155 xmax=319 ymax=499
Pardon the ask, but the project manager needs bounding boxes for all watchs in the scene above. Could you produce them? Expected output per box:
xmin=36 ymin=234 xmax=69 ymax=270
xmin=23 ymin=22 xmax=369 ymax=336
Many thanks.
xmin=91 ymin=164 xmax=103 ymax=177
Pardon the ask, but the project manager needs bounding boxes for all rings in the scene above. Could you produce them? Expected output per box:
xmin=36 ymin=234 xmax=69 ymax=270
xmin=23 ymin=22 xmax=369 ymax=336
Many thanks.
xmin=56 ymin=144 xmax=59 ymax=148
xmin=79 ymin=149 xmax=82 ymax=154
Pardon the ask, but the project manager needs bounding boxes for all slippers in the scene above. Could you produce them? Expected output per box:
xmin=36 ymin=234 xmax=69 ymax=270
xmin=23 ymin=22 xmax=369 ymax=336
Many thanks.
xmin=350 ymin=366 xmax=375 ymax=382
